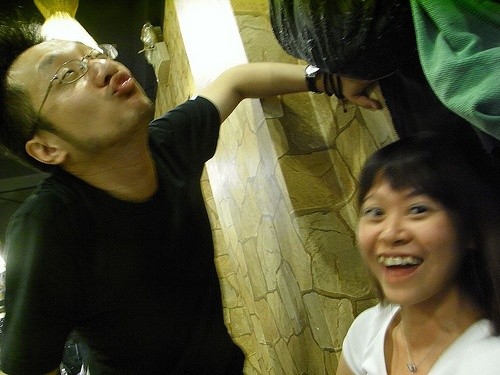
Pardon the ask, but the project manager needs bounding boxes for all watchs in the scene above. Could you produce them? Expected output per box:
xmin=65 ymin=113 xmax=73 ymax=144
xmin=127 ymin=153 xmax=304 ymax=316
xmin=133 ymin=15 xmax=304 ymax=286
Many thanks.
xmin=305 ymin=64 xmax=322 ymax=94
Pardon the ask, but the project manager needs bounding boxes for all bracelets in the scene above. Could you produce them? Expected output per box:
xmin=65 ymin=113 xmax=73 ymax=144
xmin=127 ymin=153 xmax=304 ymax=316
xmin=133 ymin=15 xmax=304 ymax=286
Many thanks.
xmin=323 ymin=72 xmax=345 ymax=100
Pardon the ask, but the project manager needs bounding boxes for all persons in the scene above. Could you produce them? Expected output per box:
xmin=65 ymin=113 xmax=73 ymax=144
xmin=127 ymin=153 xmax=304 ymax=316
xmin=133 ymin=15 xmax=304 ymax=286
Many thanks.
xmin=331 ymin=132 xmax=500 ymax=375
xmin=0 ymin=19 xmax=383 ymax=375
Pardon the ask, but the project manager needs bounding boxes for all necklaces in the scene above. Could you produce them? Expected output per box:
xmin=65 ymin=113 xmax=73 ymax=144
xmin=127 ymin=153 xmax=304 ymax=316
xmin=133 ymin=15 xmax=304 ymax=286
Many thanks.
xmin=400 ymin=320 xmax=417 ymax=372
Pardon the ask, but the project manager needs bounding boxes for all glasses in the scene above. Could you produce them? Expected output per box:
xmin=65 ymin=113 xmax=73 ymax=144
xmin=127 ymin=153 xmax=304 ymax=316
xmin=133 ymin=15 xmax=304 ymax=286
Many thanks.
xmin=36 ymin=43 xmax=119 ymax=120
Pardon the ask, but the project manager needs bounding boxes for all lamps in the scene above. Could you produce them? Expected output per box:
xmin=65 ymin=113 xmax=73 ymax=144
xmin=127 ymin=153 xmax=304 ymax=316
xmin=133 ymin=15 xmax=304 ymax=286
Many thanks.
xmin=135 ymin=20 xmax=170 ymax=84
xmin=33 ymin=0 xmax=102 ymax=53
